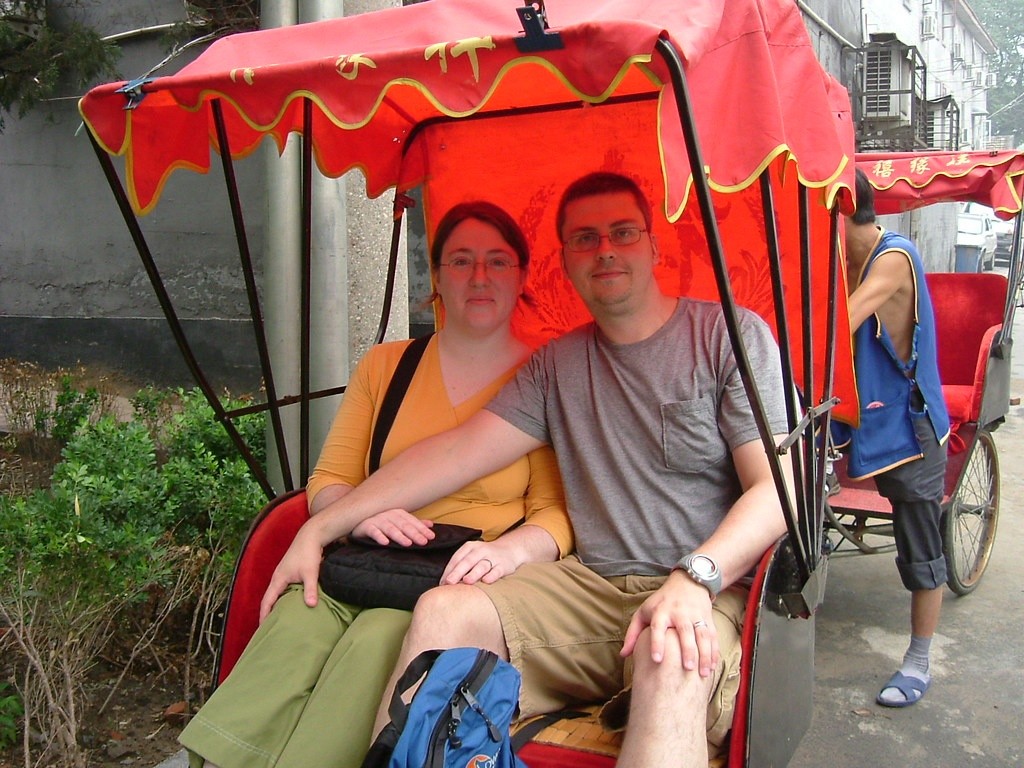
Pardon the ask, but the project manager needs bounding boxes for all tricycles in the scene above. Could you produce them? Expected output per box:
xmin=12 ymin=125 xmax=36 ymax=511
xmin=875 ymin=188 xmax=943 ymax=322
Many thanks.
xmin=78 ymin=0 xmax=858 ymax=768
xmin=823 ymin=148 xmax=1022 ymax=596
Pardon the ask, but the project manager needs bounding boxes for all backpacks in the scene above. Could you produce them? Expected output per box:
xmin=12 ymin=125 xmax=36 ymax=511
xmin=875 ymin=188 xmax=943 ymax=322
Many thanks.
xmin=362 ymin=647 xmax=529 ymax=768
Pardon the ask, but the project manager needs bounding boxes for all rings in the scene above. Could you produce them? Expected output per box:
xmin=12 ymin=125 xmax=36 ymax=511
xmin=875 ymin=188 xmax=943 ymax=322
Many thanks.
xmin=693 ymin=621 xmax=707 ymax=629
xmin=482 ymin=559 xmax=493 ymax=570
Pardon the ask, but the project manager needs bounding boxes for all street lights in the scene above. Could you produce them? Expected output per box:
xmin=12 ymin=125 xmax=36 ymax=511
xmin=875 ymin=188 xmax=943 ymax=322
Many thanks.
xmin=971 ymin=111 xmax=989 ymax=151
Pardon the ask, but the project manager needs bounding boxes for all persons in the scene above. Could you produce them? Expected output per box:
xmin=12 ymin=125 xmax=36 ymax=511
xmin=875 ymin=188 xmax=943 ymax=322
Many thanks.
xmin=178 ymin=200 xmax=572 ymax=768
xmin=259 ymin=172 xmax=807 ymax=768
xmin=815 ymin=167 xmax=950 ymax=707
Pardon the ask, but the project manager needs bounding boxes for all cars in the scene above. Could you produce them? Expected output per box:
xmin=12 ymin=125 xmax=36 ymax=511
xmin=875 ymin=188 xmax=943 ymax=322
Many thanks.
xmin=987 ymin=216 xmax=1022 ymax=264
xmin=956 ymin=213 xmax=998 ymax=273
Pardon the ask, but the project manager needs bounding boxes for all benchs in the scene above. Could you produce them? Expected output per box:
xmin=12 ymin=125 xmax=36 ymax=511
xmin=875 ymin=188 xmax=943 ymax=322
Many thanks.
xmin=215 ymin=341 xmax=813 ymax=768
xmin=822 ymin=270 xmax=1012 ymax=431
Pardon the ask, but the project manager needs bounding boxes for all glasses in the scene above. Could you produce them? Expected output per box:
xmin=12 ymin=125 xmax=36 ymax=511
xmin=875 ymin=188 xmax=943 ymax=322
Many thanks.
xmin=563 ymin=227 xmax=648 ymax=252
xmin=437 ymin=255 xmax=523 ymax=275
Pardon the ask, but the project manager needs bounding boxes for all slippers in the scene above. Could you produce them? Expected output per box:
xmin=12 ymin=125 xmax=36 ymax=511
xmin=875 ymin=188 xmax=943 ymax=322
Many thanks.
xmin=876 ymin=670 xmax=931 ymax=707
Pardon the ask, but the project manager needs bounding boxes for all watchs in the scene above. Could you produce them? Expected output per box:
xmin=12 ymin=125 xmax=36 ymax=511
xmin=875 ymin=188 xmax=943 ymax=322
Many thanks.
xmin=671 ymin=554 xmax=721 ymax=603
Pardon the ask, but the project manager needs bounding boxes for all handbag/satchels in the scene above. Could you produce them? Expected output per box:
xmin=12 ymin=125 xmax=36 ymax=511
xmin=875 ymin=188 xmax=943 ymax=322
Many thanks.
xmin=317 ymin=523 xmax=483 ymax=612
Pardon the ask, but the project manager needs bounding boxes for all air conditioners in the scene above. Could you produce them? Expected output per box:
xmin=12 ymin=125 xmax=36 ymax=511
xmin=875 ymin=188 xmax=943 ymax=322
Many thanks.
xmin=862 ymin=42 xmax=914 ymax=129
xmin=966 ymin=64 xmax=975 ymax=79
xmin=923 ymin=15 xmax=937 ymax=35
xmin=961 ymin=128 xmax=971 ymax=142
xmin=917 ymin=96 xmax=957 ymax=154
xmin=976 ymin=71 xmax=985 ymax=86
xmin=985 ymin=72 xmax=996 ymax=91
xmin=954 ymin=42 xmax=964 ymax=59
